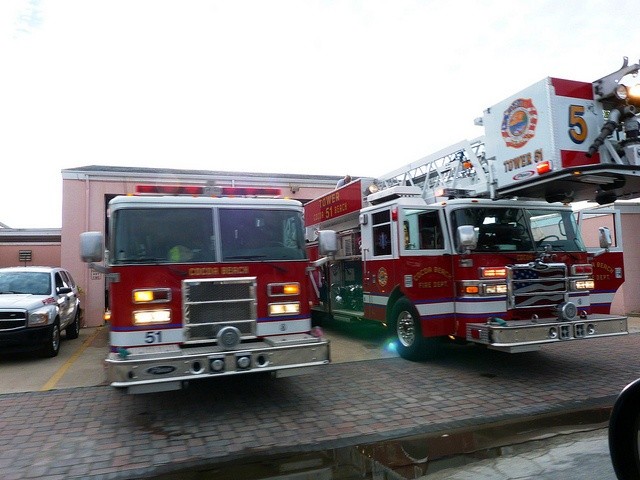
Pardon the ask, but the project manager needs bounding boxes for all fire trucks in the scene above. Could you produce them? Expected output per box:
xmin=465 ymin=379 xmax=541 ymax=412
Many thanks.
xmin=80 ymin=179 xmax=331 ymax=396
xmin=307 ymin=55 xmax=639 ymax=362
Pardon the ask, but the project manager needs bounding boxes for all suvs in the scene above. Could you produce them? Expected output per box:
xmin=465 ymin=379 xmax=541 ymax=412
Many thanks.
xmin=0 ymin=266 xmax=81 ymax=358
